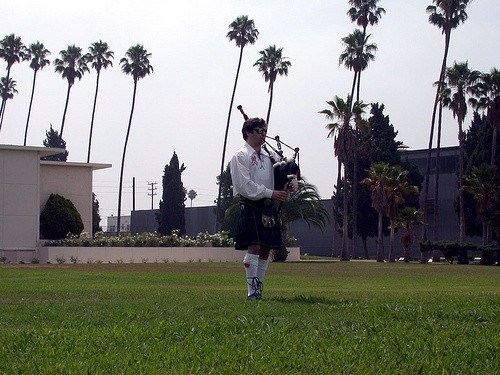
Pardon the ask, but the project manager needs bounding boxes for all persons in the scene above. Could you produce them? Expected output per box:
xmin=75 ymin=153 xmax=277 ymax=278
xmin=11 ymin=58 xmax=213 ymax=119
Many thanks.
xmin=230 ymin=119 xmax=298 ymax=301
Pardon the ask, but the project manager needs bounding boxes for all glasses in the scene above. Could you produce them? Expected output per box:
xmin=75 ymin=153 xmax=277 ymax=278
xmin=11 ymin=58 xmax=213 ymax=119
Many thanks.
xmin=253 ymin=127 xmax=267 ymax=134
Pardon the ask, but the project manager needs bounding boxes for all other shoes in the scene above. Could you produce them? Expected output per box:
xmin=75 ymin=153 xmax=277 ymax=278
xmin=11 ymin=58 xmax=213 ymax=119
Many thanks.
xmin=248 ymin=292 xmax=261 ymax=300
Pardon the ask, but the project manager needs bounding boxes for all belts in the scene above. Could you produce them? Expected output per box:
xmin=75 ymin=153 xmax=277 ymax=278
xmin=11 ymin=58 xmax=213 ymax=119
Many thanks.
xmin=240 ymin=195 xmax=273 ymax=203
